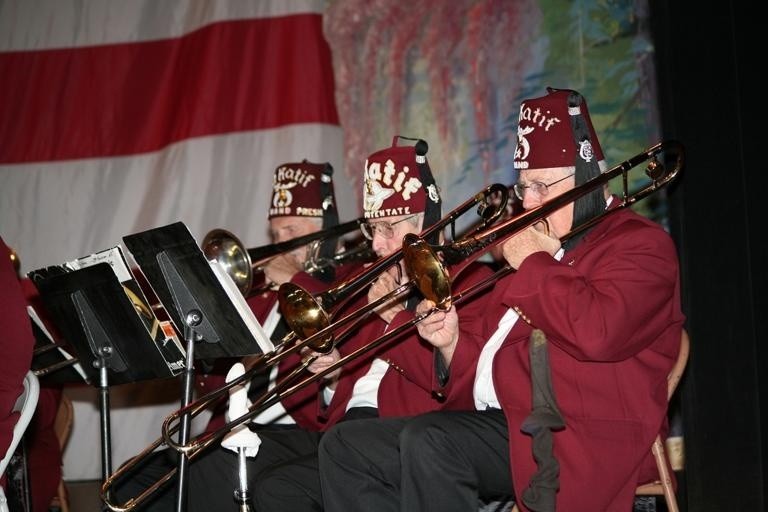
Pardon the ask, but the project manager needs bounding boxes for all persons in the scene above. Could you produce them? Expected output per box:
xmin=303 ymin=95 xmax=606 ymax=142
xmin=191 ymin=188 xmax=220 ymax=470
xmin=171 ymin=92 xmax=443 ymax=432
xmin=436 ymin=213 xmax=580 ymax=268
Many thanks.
xmin=0 ymin=236 xmax=62 ymax=512
xmin=109 ymin=158 xmax=366 ymax=512
xmin=184 ymin=146 xmax=496 ymax=512
xmin=318 ymin=87 xmax=686 ymax=512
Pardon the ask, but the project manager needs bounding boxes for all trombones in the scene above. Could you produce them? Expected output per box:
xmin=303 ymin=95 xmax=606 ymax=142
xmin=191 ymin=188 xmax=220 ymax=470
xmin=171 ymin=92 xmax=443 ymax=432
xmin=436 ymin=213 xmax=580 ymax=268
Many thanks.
xmin=162 ymin=136 xmax=694 ymax=452
xmin=99 ymin=184 xmax=508 ymax=511
xmin=198 ymin=213 xmax=378 ymax=302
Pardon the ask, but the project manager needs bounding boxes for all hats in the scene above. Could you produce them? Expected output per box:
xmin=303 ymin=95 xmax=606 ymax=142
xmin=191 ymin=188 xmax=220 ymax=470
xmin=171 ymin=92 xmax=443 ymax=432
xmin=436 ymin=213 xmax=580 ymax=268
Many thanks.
xmin=363 ymin=145 xmax=441 ymax=218
xmin=513 ymin=90 xmax=605 ymax=170
xmin=268 ymin=161 xmax=338 ymax=218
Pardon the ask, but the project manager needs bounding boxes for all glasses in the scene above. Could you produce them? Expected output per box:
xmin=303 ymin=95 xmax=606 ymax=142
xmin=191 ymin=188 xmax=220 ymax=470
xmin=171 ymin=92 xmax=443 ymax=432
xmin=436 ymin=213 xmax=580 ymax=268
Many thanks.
xmin=514 ymin=169 xmax=575 ymax=201
xmin=361 ymin=212 xmax=417 ymax=240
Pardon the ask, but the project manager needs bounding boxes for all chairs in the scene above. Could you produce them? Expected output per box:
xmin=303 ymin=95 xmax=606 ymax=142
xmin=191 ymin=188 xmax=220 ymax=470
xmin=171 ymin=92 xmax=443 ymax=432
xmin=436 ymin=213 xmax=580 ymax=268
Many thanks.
xmin=630 ymin=327 xmax=693 ymax=511
xmin=0 ymin=367 xmax=77 ymax=512
